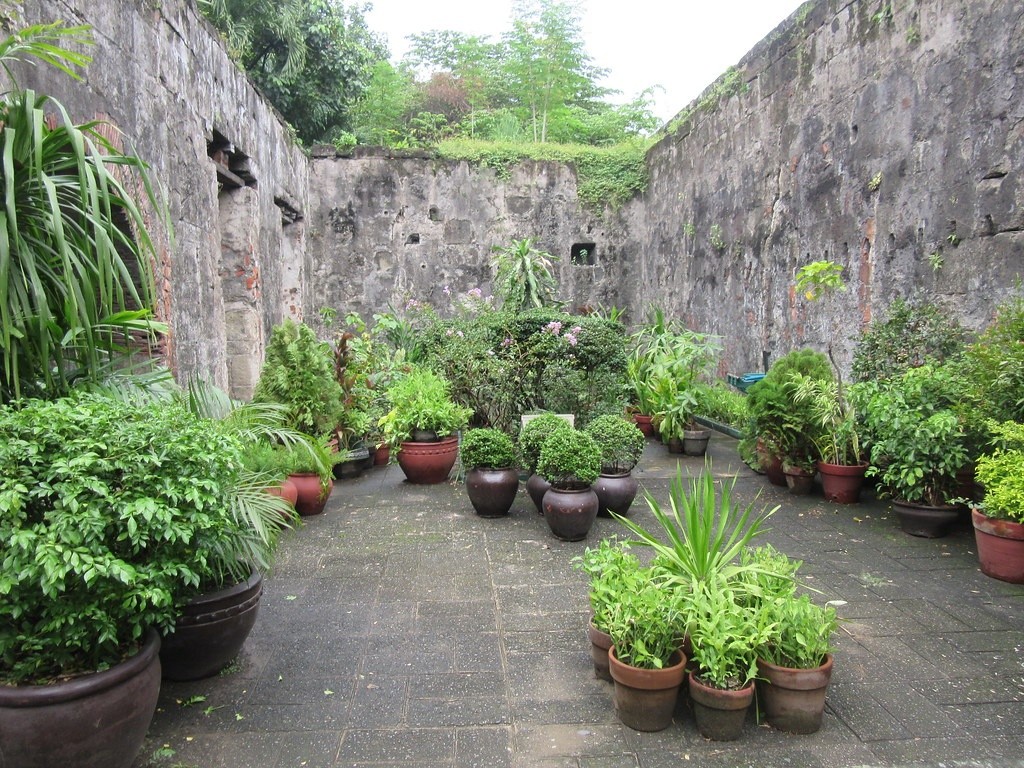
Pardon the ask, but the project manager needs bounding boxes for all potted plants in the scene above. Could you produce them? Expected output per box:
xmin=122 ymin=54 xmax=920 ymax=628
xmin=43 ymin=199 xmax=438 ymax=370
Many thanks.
xmin=0 ymin=86 xmax=1024 ymax=768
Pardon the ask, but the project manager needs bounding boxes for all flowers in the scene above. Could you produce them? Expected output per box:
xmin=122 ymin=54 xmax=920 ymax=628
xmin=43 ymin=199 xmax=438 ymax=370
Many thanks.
xmin=419 ymin=285 xmax=582 ymax=413
